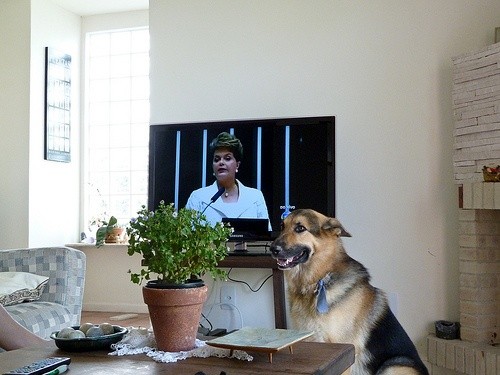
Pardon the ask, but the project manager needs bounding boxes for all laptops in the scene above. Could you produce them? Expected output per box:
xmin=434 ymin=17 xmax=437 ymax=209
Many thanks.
xmin=222 ymin=218 xmax=269 ymax=241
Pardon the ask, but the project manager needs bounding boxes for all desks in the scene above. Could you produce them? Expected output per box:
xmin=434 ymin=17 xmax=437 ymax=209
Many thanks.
xmin=0 ymin=335 xmax=356 ymax=375
xmin=141 ymin=252 xmax=288 ymax=330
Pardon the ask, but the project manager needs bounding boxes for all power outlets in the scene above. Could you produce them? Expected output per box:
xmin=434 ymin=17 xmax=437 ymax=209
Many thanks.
xmin=218 ymin=285 xmax=237 ymax=311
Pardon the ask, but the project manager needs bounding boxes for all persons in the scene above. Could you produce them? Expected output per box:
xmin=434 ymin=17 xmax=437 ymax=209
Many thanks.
xmin=0 ymin=303 xmax=49 ymax=351
xmin=186 ymin=132 xmax=273 ymax=231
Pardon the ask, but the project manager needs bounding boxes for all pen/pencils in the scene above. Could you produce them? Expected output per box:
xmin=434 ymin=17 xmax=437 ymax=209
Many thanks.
xmin=42 ymin=364 xmax=68 ymax=375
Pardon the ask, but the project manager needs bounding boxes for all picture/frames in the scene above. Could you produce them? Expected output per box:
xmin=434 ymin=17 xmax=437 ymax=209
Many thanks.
xmin=44 ymin=45 xmax=73 ymax=163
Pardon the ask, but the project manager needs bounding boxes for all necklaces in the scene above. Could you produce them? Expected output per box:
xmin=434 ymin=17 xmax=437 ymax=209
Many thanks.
xmin=218 ymin=185 xmax=235 ymax=197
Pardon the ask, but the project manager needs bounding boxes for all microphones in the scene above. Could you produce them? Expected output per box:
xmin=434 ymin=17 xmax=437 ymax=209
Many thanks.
xmin=197 ymin=187 xmax=225 ymax=219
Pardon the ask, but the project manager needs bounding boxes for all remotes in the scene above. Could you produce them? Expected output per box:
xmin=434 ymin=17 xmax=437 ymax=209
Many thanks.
xmin=2 ymin=357 xmax=71 ymax=375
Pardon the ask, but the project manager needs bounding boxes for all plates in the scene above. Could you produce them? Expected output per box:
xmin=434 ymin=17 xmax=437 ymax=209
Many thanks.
xmin=50 ymin=324 xmax=128 ymax=353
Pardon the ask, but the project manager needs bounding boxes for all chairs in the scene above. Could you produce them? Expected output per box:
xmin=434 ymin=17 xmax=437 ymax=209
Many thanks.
xmin=0 ymin=245 xmax=87 ymax=354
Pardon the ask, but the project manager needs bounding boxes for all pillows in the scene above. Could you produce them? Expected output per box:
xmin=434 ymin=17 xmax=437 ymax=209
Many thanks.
xmin=0 ymin=271 xmax=50 ymax=307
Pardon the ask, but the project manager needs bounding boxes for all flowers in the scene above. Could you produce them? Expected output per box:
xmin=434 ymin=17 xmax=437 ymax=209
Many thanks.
xmin=125 ymin=200 xmax=235 ymax=286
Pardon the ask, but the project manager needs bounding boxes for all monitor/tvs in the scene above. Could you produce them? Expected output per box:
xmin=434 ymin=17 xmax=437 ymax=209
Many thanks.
xmin=147 ymin=115 xmax=336 ymax=240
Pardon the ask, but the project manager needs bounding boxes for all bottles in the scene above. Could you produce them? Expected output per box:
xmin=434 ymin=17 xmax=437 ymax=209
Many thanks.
xmin=281 ymin=205 xmax=292 ymax=219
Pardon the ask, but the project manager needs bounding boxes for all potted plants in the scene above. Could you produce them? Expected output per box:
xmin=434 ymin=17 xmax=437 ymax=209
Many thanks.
xmin=95 ymin=215 xmax=123 ymax=249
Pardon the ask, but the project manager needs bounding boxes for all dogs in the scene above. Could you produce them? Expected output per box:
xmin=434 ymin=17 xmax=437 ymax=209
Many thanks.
xmin=269 ymin=208 xmax=429 ymax=375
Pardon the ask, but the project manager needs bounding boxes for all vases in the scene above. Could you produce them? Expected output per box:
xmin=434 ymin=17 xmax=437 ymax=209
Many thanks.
xmin=141 ymin=279 xmax=209 ymax=354
